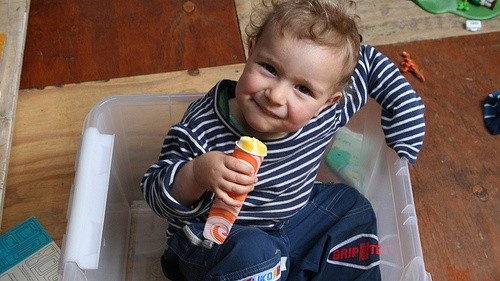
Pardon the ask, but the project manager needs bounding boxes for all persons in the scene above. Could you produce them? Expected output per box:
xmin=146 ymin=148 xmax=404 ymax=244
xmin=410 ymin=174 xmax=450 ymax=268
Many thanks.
xmin=142 ymin=0 xmax=424 ymax=281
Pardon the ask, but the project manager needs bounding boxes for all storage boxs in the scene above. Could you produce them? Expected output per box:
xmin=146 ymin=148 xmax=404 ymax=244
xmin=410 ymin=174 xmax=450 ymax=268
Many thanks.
xmin=58 ymin=90 xmax=432 ymax=281
